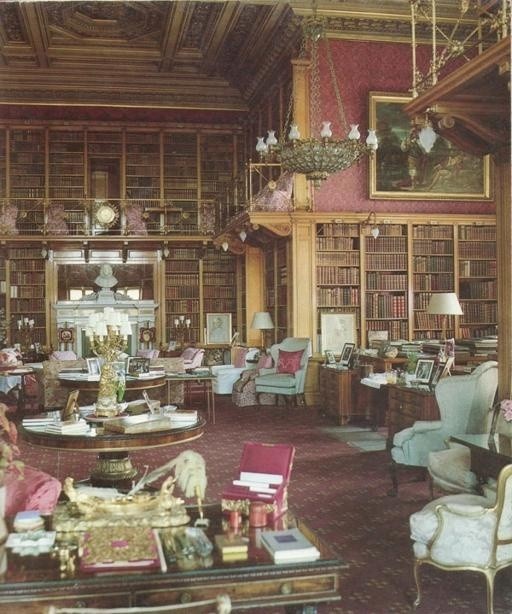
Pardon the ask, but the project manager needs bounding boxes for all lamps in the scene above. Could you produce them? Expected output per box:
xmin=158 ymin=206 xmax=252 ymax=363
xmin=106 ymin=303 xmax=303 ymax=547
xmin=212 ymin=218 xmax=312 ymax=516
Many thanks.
xmin=174 ymin=314 xmax=193 ymax=344
xmin=254 ymin=0 xmax=379 ymax=184
xmin=16 ymin=315 xmax=35 ymax=340
xmin=361 ymin=209 xmax=382 ymax=241
xmin=251 ymin=310 xmax=275 ymax=352
xmin=84 ymin=306 xmax=131 ymax=413
xmin=424 ymin=290 xmax=465 ymax=347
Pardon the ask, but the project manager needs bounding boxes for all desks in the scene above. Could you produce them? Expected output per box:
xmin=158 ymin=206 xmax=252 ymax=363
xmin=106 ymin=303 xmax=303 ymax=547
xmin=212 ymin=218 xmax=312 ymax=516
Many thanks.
xmin=363 ymin=374 xmax=438 ymax=448
xmin=166 ymin=368 xmax=219 ymax=426
xmin=361 ymin=352 xmax=408 ymax=373
xmin=21 ymin=406 xmax=206 ymax=492
xmin=246 ymin=358 xmax=258 ymax=364
xmin=1 ymin=492 xmax=351 ymax=614
xmin=57 ymin=368 xmax=167 ymax=390
xmin=448 ymin=430 xmax=511 ymax=475
xmin=1 ymin=364 xmax=45 ymax=425
xmin=317 ymin=363 xmax=380 ymax=430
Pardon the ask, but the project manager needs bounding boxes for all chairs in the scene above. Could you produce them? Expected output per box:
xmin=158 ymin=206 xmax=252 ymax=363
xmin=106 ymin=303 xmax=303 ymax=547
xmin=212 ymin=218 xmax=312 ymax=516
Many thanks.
xmin=407 ymin=462 xmax=512 ymax=613
xmin=425 ymin=403 xmax=512 ymax=502
xmin=31 ymin=350 xmax=88 ymax=409
xmin=385 ymin=359 xmax=500 ymax=497
xmin=253 ymin=334 xmax=312 ymax=414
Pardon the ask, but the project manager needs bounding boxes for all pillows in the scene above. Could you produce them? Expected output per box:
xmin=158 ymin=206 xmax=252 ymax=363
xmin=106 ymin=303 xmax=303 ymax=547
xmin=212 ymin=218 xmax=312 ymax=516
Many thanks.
xmin=234 ymin=348 xmax=249 ymax=368
xmin=276 ymin=348 xmax=306 ymax=374
xmin=257 ymin=354 xmax=272 ymax=375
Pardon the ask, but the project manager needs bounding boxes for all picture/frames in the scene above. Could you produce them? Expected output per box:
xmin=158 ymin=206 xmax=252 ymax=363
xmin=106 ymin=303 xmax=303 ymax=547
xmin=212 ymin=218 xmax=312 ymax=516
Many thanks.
xmin=319 ymin=310 xmax=358 ymax=356
xmin=366 ymin=85 xmax=498 ymax=205
xmin=205 ymin=311 xmax=233 ymax=344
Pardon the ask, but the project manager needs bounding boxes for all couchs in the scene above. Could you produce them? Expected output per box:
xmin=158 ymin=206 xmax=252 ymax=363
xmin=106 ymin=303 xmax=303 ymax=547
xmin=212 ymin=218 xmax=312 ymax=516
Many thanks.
xmin=231 ymin=354 xmax=297 ymax=408
xmin=154 ymin=347 xmax=206 ymax=405
xmin=208 ymin=345 xmax=261 ymax=395
xmin=0 ymin=400 xmax=65 ymax=518
xmin=136 ymin=348 xmax=160 ymax=364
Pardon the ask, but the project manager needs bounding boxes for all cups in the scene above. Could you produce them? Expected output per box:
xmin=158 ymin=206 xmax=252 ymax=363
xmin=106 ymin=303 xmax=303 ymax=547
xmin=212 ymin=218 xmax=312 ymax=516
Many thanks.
xmin=394 ymin=369 xmax=407 ymax=385
xmin=385 ymin=368 xmax=396 ymax=384
xmin=221 ymin=498 xmax=249 ymax=544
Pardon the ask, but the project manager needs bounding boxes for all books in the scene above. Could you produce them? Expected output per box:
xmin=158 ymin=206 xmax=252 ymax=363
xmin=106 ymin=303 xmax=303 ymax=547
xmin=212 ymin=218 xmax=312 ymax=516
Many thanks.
xmin=263 ymin=243 xmax=287 ymax=346
xmin=166 ymin=243 xmax=238 ymax=347
xmin=221 ymin=552 xmax=249 ymax=562
xmin=59 ymin=355 xmax=166 ymax=382
xmin=0 ymin=244 xmax=47 ymax=351
xmin=8 ymin=126 xmax=242 ymax=235
xmin=316 ymin=219 xmax=497 ymax=363
xmin=213 ymin=533 xmax=248 ymax=554
xmin=259 ymin=526 xmax=322 ymax=564
xmin=21 ymin=395 xmax=198 ymax=436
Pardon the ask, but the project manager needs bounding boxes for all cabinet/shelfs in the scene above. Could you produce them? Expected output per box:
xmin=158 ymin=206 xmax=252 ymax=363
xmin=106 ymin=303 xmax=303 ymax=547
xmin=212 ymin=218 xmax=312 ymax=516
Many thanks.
xmin=160 ymin=247 xmax=264 ymax=347
xmin=262 ymin=238 xmax=289 ymax=349
xmin=0 ymin=122 xmax=247 ymax=236
xmin=311 ymin=218 xmax=498 ymax=354
xmin=0 ymin=248 xmax=50 ymax=345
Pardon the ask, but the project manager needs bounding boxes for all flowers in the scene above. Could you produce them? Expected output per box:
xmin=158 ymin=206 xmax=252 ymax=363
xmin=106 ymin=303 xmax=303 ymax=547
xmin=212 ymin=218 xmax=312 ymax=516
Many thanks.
xmin=500 ymin=399 xmax=512 ymax=422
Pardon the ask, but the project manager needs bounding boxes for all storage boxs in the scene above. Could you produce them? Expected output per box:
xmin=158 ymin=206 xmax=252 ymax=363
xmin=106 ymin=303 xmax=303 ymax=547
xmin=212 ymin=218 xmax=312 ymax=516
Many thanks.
xmin=216 ymin=437 xmax=295 ymax=521
